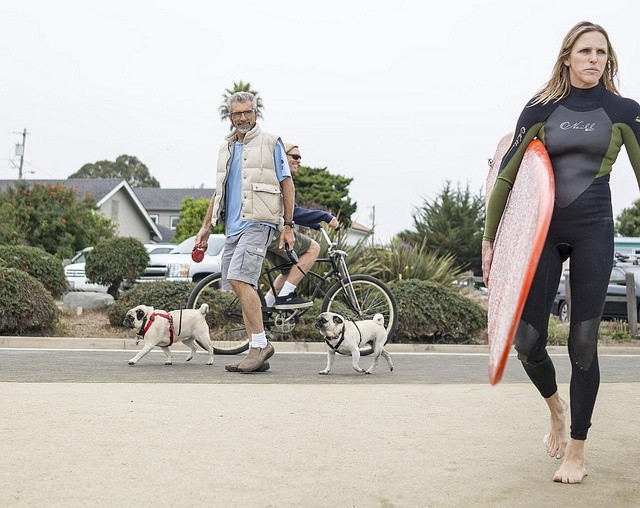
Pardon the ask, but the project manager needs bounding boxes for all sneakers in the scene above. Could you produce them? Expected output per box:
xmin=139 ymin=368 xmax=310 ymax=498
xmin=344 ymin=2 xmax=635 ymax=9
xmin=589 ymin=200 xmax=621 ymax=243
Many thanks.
xmin=274 ymin=291 xmax=313 ymax=309
xmin=237 ymin=339 xmax=275 ymax=371
xmin=226 ymin=356 xmax=270 ymax=371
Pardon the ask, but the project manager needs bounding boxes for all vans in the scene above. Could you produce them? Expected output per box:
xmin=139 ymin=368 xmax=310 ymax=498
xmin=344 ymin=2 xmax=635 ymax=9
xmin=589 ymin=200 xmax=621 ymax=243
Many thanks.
xmin=166 ymin=233 xmax=226 ymax=290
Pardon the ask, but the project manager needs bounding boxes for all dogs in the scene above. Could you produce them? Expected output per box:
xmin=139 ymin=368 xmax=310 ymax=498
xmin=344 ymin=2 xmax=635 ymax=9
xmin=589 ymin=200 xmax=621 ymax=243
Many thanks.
xmin=123 ymin=303 xmax=214 ymax=364
xmin=315 ymin=311 xmax=393 ymax=373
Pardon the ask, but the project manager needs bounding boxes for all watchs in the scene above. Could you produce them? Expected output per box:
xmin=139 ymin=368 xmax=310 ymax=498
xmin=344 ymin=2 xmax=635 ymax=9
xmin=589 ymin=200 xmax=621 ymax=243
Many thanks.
xmin=285 ymin=221 xmax=295 ymax=227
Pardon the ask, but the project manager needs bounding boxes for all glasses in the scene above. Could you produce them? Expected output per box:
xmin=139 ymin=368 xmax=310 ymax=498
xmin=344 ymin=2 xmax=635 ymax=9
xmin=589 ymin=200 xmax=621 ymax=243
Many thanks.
xmin=287 ymin=153 xmax=301 ymax=160
xmin=232 ymin=110 xmax=253 ymax=120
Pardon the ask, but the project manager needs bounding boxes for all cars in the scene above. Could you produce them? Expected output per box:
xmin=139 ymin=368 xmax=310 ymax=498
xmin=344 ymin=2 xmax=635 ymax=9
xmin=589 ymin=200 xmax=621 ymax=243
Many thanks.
xmin=549 ymin=265 xmax=640 ymax=323
xmin=62 ymin=247 xmax=123 ymax=292
xmin=121 ymin=244 xmax=177 ymax=292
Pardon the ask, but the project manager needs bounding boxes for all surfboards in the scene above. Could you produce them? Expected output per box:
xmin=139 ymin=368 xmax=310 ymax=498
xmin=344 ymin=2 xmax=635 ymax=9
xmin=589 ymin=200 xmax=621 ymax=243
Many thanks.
xmin=487 ymin=133 xmax=556 ymax=385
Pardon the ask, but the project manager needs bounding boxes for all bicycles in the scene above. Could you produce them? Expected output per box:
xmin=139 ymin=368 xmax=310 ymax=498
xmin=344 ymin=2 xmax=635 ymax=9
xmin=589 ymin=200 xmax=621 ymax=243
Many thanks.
xmin=187 ymin=220 xmax=398 ymax=356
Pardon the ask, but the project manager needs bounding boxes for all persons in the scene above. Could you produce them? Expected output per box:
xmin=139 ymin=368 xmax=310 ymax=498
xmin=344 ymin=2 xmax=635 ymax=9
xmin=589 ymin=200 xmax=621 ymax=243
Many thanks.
xmin=481 ymin=22 xmax=639 ymax=485
xmin=259 ymin=144 xmax=339 ymax=338
xmin=196 ymin=91 xmax=295 ymax=373
